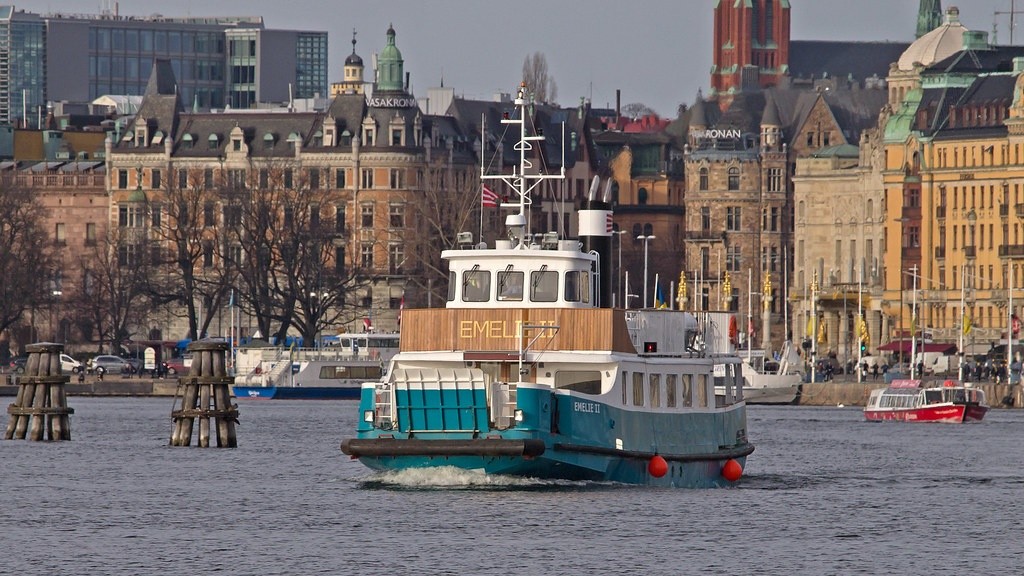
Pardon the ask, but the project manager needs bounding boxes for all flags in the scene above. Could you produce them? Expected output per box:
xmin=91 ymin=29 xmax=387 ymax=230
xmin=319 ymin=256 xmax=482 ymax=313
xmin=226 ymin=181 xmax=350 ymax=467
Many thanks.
xmin=963 ymin=315 xmax=972 ymax=335
xmin=483 ymin=185 xmax=499 ymax=208
xmin=1012 ymin=314 xmax=1020 ymax=335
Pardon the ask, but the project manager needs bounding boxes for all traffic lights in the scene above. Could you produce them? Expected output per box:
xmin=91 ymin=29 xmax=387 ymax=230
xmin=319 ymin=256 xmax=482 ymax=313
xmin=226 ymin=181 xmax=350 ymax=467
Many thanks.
xmin=862 ymin=342 xmax=867 ymax=352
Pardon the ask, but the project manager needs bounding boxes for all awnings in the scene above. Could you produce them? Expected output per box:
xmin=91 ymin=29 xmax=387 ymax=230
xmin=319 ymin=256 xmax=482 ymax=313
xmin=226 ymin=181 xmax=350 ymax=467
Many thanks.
xmin=876 ymin=340 xmax=956 ymax=352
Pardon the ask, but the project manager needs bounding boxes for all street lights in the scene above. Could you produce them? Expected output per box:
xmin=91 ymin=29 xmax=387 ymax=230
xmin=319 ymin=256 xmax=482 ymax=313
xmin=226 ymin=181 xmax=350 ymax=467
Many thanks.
xmin=748 ymin=265 xmax=764 ymax=367
xmin=625 ymin=271 xmax=640 ymax=310
xmin=637 ymin=234 xmax=657 ymax=308
xmin=612 ymin=230 xmax=627 ymax=308
xmin=810 ymin=267 xmax=828 ymax=384
xmin=693 ymin=269 xmax=709 ymax=318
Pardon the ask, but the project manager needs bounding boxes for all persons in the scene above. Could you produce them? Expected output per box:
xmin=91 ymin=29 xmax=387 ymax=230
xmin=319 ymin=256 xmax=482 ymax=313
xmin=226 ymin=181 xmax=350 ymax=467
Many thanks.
xmin=816 ymin=361 xmax=833 ymax=382
xmin=863 ymin=360 xmax=889 ymax=381
xmin=805 ymin=362 xmax=811 ymax=383
xmin=963 ymin=361 xmax=1006 ymax=383
xmin=917 ymin=360 xmax=925 ymax=379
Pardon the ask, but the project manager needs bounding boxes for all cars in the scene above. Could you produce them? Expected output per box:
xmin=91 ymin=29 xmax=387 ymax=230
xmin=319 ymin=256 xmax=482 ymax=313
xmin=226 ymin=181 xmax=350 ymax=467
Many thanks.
xmin=887 ymin=361 xmax=911 ymax=375
xmin=165 ymin=356 xmax=191 ymax=375
xmin=9 ymin=358 xmax=27 ymax=374
xmin=60 ymin=354 xmax=84 ymax=373
xmin=127 ymin=358 xmax=144 ymax=371
xmin=91 ymin=355 xmax=133 ymax=373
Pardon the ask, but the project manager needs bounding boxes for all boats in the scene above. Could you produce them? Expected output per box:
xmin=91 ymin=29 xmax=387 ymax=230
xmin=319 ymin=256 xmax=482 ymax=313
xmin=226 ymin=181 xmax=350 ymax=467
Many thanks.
xmin=863 ymin=378 xmax=993 ymax=425
xmin=230 ymin=353 xmax=384 ymax=400
xmin=338 ymin=79 xmax=759 ymax=488
xmin=712 ymin=339 xmax=804 ymax=405
